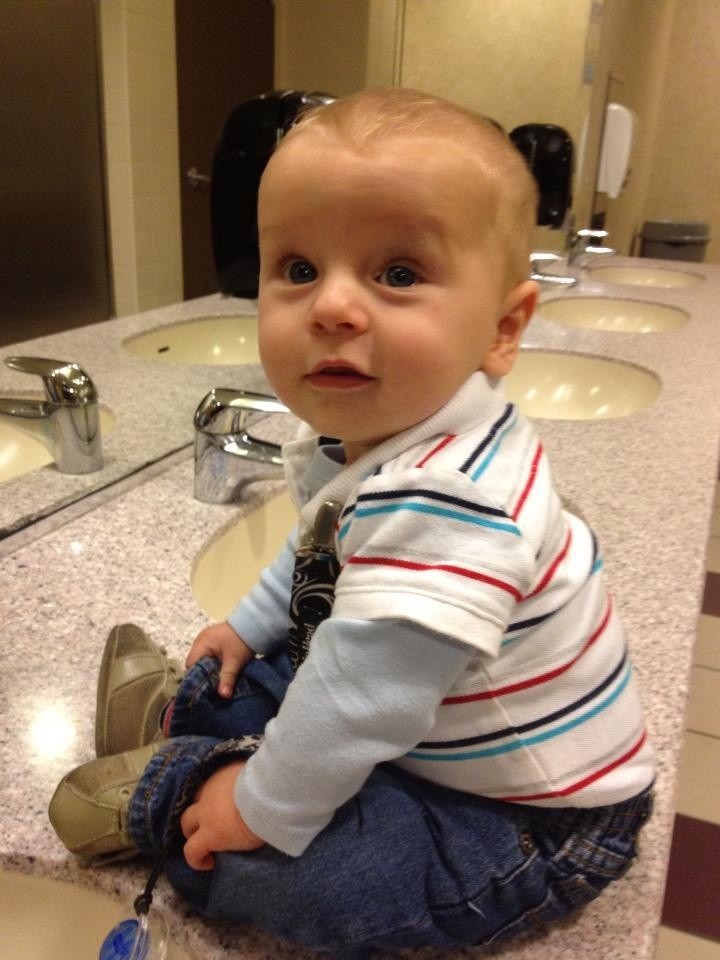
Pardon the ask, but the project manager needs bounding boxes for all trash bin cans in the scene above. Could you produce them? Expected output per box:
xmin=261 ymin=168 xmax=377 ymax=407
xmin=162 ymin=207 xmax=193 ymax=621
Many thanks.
xmin=638 ymin=219 xmax=711 ymax=262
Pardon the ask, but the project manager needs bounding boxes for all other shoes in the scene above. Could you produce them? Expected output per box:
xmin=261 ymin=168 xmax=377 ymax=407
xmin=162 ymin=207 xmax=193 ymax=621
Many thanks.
xmin=94 ymin=625 xmax=185 ymax=759
xmin=49 ymin=737 xmax=180 ymax=858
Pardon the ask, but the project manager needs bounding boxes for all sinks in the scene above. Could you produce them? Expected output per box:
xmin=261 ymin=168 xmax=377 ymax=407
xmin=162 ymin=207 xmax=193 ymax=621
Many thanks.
xmin=496 ymin=344 xmax=666 ymax=423
xmin=0 ymin=387 xmax=120 ymax=484
xmin=588 ymin=263 xmax=710 ymax=291
xmin=2 ymin=845 xmax=218 ymax=960
xmin=189 ymin=480 xmax=599 ymax=627
xmin=535 ymin=291 xmax=692 ymax=334
xmin=120 ymin=311 xmax=259 ymax=367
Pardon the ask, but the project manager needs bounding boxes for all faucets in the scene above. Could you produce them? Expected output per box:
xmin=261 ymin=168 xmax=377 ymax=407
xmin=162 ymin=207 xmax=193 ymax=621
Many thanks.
xmin=568 ymin=229 xmax=614 ymax=269
xmin=527 ymin=250 xmax=579 ymax=290
xmin=2 ymin=356 xmax=106 ymax=476
xmin=189 ymin=386 xmax=296 ymax=502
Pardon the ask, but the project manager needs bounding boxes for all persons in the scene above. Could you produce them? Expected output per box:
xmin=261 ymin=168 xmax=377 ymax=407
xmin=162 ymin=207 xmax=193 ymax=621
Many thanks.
xmin=44 ymin=84 xmax=660 ymax=960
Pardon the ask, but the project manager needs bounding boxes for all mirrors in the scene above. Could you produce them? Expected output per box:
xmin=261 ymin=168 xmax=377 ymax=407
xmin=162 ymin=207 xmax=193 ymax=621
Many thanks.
xmin=0 ymin=0 xmax=415 ymax=546
